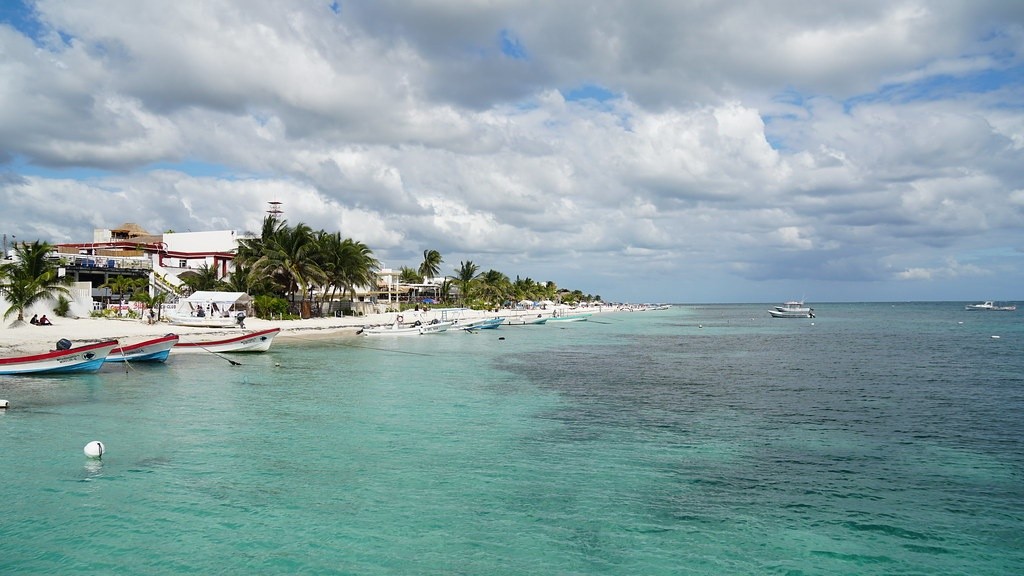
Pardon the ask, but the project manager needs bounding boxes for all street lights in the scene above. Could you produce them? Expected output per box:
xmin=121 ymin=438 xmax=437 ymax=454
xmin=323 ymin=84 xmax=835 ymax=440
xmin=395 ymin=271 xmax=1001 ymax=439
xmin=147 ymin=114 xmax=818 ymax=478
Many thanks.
xmin=119 ymin=289 xmax=125 ymax=318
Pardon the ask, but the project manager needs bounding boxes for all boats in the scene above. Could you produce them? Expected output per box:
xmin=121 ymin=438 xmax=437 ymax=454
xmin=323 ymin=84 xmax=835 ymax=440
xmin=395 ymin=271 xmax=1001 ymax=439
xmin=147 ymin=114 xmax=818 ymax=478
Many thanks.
xmin=768 ymin=301 xmax=816 ymax=318
xmin=0 ymin=339 xmax=118 ymax=374
xmin=165 ymin=300 xmax=238 ymax=328
xmin=170 ymin=328 xmax=281 ymax=353
xmin=106 ymin=334 xmax=179 ymax=364
xmin=362 ymin=313 xmax=591 ymax=335
xmin=622 ymin=305 xmax=672 ymax=311
xmin=966 ymin=301 xmax=1016 ymax=311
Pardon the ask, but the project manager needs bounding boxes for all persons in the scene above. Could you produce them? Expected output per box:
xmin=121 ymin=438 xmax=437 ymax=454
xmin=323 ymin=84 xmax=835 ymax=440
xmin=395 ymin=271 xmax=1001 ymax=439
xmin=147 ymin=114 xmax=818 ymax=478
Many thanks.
xmin=147 ymin=307 xmax=154 ymax=324
xmin=40 ymin=314 xmax=53 ymax=325
xmin=30 ymin=314 xmax=39 ymax=326
xmin=190 ymin=302 xmax=220 ymax=317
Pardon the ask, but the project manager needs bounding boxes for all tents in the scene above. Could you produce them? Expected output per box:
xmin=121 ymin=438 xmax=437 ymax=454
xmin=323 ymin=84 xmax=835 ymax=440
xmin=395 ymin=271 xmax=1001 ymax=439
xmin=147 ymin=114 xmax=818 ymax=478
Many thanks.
xmin=184 ymin=291 xmax=253 ymax=317
